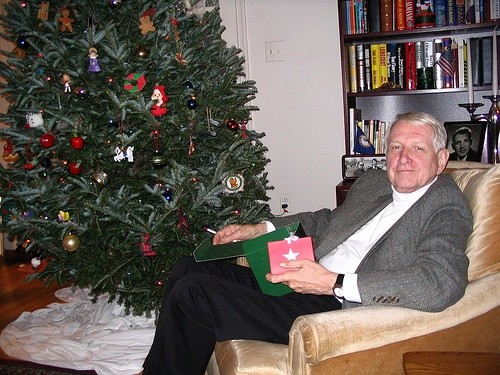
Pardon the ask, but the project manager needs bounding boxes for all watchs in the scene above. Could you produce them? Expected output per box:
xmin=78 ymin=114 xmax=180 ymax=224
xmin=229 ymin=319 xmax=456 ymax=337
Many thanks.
xmin=333 ymin=274 xmax=345 ymax=300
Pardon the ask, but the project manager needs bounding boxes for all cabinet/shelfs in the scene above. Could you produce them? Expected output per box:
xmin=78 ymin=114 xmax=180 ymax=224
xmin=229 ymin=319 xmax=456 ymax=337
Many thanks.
xmin=336 ymin=0 xmax=500 ymax=156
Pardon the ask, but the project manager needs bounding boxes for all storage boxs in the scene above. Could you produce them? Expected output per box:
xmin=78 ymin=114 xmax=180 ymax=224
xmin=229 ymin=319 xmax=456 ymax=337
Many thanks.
xmin=193 ymin=221 xmax=315 ymax=296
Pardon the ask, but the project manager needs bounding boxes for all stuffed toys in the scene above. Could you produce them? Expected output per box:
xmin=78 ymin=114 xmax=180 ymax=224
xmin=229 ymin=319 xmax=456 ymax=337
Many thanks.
xmin=24 ymin=113 xmax=44 ymax=129
xmin=150 ymin=84 xmax=168 ymax=115
xmin=31 ymin=257 xmax=41 ymax=270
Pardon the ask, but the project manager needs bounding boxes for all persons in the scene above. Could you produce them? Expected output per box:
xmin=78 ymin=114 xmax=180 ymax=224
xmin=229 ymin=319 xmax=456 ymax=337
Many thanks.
xmin=448 ymin=127 xmax=480 ymax=162
xmin=88 ymin=47 xmax=101 ymax=72
xmin=135 ymin=112 xmax=473 ymax=375
xmin=62 ymin=74 xmax=71 ymax=93
xmin=353 ymin=159 xmax=381 ymax=176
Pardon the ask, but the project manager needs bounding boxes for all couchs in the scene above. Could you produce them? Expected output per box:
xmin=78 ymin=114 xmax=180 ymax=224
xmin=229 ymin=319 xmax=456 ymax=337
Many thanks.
xmin=206 ymin=163 xmax=499 ymax=375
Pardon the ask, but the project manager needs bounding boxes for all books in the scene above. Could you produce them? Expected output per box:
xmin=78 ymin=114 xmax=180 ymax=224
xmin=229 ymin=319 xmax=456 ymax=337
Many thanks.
xmin=347 ymin=0 xmax=500 ymax=34
xmin=350 ymin=108 xmax=390 ymax=155
xmin=348 ymin=34 xmax=500 ymax=93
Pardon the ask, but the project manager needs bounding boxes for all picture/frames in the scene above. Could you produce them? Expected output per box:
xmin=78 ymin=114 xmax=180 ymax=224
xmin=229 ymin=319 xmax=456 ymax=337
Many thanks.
xmin=442 ymin=121 xmax=487 ymax=162
xmin=341 ymin=156 xmax=387 ymax=180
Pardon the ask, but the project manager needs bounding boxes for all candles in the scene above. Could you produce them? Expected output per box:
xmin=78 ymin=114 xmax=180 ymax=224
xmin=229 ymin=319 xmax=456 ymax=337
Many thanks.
xmin=492 ymin=25 xmax=498 ymax=95
xmin=466 ymin=38 xmax=473 ymax=103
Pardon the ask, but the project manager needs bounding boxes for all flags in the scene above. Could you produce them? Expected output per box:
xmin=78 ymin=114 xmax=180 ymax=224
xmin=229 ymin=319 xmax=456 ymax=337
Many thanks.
xmin=436 ymin=48 xmax=455 ymax=78
xmin=354 ymin=126 xmax=375 ymax=155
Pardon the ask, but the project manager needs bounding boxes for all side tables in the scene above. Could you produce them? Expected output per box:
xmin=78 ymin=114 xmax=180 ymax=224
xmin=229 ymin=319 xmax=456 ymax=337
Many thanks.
xmin=402 ymin=352 xmax=500 ymax=375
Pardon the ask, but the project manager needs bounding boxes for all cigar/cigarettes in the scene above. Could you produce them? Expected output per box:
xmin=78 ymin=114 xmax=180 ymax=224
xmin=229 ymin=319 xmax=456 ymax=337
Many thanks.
xmin=207 ymin=229 xmax=216 ymax=234
